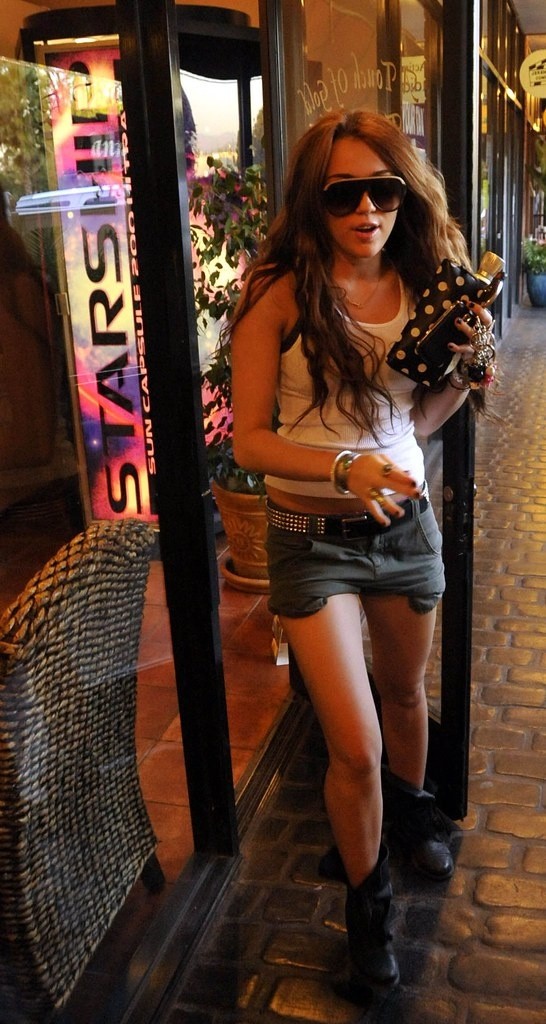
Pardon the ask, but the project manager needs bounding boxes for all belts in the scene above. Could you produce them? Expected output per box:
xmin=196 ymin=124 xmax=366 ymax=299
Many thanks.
xmin=263 ymin=479 xmax=429 ymax=541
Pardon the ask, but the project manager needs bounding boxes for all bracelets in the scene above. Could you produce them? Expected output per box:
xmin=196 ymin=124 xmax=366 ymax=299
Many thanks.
xmin=447 ymin=368 xmax=472 ymax=391
xmin=330 ymin=450 xmax=361 ymax=495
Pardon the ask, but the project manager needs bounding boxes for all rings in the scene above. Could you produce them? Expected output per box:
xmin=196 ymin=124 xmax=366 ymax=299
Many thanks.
xmin=382 ymin=464 xmax=395 ymax=476
xmin=369 ymin=488 xmax=380 ymax=498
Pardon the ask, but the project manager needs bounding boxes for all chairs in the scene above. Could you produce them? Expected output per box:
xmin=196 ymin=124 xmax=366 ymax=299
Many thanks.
xmin=2 ymin=517 xmax=171 ymax=1023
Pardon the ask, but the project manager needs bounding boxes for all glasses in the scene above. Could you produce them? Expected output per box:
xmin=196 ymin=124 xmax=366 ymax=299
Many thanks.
xmin=323 ymin=175 xmax=408 ymax=217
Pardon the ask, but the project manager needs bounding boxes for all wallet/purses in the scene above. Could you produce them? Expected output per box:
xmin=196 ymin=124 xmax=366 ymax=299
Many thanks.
xmin=386 ymin=259 xmax=494 ymax=395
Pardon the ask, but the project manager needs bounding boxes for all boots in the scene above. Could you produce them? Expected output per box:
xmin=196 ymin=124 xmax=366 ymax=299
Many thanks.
xmin=317 ymin=841 xmax=401 ymax=1008
xmin=383 ymin=767 xmax=454 ymax=879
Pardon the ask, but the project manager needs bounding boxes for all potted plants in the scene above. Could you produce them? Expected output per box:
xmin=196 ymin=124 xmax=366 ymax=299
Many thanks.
xmin=183 ymin=150 xmax=279 ymax=596
xmin=521 ymin=236 xmax=546 ymax=308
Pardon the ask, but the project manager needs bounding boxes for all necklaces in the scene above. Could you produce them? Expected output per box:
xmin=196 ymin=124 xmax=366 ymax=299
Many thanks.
xmin=325 ymin=260 xmax=382 ymax=309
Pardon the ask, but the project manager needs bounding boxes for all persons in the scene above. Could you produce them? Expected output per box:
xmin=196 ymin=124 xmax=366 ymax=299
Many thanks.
xmin=0 ymin=184 xmax=66 ymax=608
xmin=229 ymin=108 xmax=503 ymax=995
